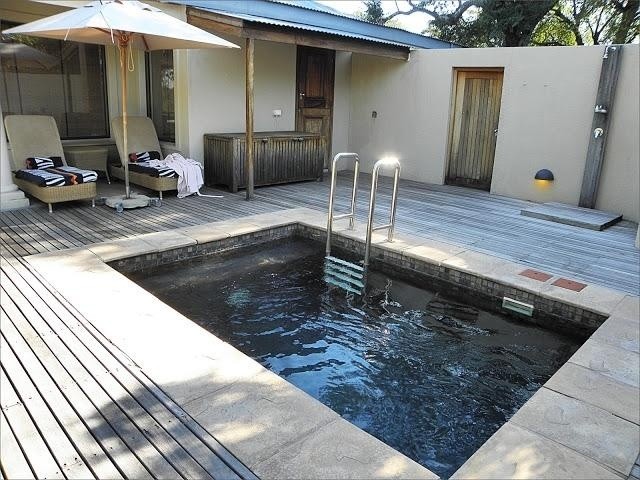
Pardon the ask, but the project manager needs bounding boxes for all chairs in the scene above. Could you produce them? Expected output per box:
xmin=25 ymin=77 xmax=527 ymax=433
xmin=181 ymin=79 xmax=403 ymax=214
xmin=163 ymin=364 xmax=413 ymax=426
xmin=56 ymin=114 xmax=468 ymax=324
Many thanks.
xmin=4 ymin=112 xmax=202 ymax=215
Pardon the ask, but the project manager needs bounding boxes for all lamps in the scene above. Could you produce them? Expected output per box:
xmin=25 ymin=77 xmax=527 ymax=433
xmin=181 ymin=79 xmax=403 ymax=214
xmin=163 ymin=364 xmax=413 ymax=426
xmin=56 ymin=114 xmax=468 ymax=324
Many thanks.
xmin=533 ymin=169 xmax=555 ymax=183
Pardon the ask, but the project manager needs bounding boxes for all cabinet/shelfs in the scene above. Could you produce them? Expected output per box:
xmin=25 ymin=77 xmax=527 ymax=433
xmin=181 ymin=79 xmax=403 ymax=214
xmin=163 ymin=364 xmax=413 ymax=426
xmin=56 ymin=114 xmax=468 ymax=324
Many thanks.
xmin=204 ymin=129 xmax=323 ymax=194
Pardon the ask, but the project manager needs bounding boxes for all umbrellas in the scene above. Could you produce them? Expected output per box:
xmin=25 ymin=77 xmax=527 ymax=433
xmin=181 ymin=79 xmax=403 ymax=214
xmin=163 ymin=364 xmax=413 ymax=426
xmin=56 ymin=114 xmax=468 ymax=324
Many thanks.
xmin=0 ymin=1 xmax=244 ymax=199
